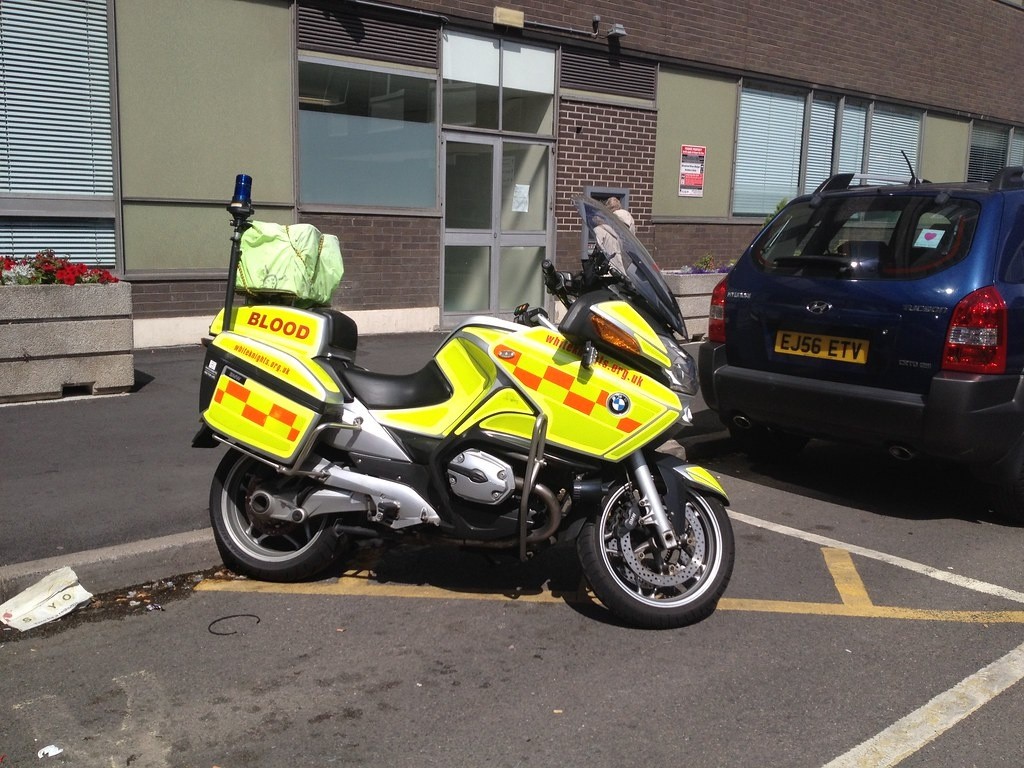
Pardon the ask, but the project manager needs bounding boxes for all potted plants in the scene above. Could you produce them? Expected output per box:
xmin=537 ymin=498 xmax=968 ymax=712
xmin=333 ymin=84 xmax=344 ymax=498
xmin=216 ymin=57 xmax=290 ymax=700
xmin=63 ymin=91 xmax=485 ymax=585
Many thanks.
xmin=0 ymin=278 xmax=136 ymax=405
xmin=657 ymin=261 xmax=733 ymax=341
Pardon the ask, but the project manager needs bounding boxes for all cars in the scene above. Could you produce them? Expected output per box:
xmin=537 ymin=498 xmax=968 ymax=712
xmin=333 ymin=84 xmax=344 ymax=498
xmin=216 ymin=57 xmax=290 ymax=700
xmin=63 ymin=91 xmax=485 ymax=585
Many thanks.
xmin=700 ymin=164 xmax=1023 ymax=501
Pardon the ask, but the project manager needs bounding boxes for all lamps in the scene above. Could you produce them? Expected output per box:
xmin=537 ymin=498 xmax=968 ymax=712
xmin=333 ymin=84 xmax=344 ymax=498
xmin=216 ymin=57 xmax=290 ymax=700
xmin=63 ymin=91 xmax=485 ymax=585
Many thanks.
xmin=608 ymin=23 xmax=628 ymax=39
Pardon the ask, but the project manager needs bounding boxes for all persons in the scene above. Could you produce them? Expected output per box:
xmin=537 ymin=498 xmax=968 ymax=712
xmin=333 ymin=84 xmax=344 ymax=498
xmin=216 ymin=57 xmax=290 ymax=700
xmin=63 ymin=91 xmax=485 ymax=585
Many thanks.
xmin=605 ymin=196 xmax=634 ymax=236
xmin=591 ymin=214 xmax=626 ymax=277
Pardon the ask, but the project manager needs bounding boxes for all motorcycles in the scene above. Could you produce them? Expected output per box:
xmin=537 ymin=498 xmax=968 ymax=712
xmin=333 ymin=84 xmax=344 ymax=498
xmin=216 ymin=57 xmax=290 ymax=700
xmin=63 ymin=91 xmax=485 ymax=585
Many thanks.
xmin=189 ymin=174 xmax=735 ymax=628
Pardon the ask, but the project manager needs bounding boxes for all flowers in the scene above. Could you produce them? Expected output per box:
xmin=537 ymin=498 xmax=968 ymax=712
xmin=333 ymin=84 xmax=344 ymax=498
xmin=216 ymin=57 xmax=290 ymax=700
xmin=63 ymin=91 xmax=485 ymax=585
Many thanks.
xmin=0 ymin=247 xmax=121 ymax=287
xmin=682 ymin=254 xmax=731 ymax=275
